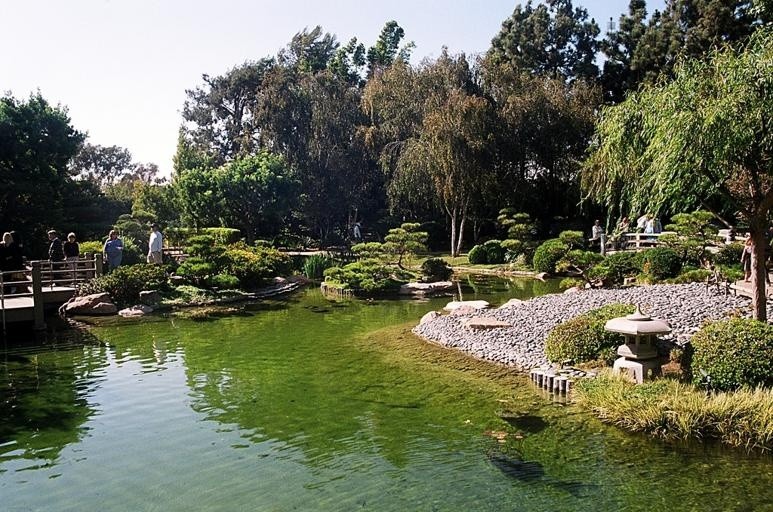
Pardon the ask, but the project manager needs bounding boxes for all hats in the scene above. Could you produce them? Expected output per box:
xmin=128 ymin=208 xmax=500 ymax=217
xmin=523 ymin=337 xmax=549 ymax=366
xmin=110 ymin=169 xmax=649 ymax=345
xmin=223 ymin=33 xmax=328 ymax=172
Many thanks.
xmin=150 ymin=223 xmax=157 ymax=228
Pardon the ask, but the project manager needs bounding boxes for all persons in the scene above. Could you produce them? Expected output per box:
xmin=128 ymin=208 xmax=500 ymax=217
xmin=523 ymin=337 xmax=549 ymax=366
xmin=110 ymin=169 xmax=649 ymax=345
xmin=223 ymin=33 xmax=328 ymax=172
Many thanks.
xmin=0 ymin=232 xmax=12 ymax=293
xmin=591 ymin=212 xmax=663 ymax=241
xmin=47 ymin=230 xmax=62 ymax=286
xmin=145 ymin=223 xmax=162 ymax=264
xmin=101 ymin=229 xmax=123 ymax=273
xmin=61 ymin=231 xmax=78 ymax=284
